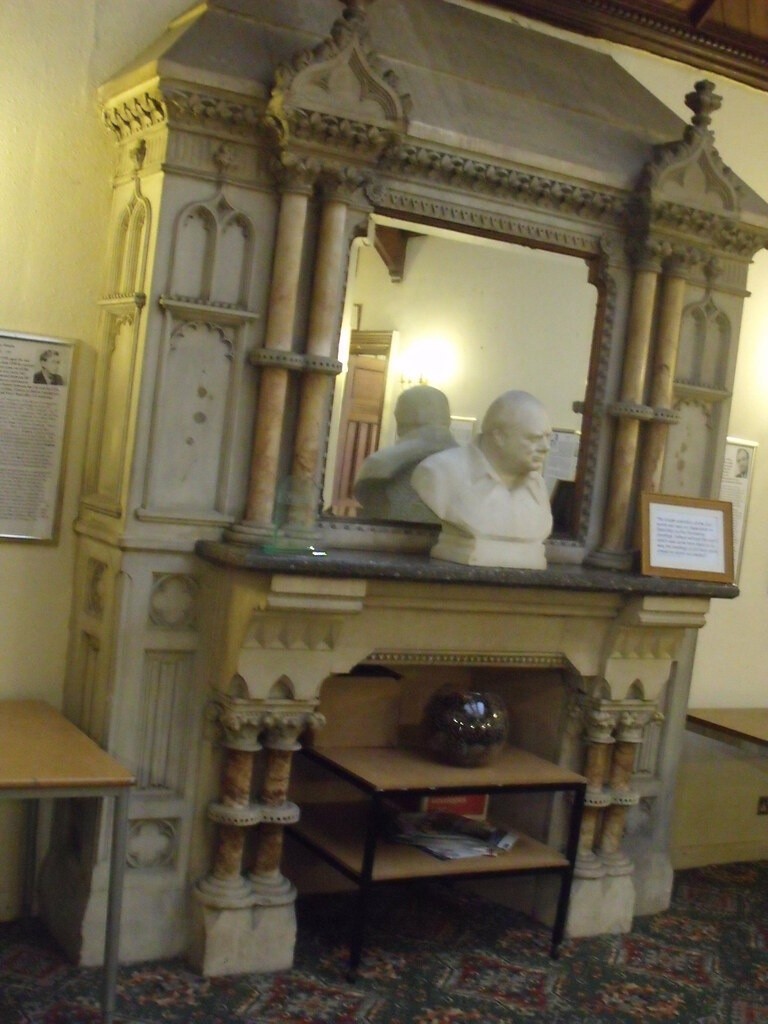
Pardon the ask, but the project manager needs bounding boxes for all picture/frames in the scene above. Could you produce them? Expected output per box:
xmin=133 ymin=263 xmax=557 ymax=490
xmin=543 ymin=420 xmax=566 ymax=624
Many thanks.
xmin=0 ymin=329 xmax=78 ymax=547
xmin=637 ymin=490 xmax=736 ymax=584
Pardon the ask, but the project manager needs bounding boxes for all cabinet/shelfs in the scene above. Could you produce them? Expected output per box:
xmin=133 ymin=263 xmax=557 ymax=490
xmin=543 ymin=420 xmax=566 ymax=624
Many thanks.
xmin=297 ymin=744 xmax=590 ymax=983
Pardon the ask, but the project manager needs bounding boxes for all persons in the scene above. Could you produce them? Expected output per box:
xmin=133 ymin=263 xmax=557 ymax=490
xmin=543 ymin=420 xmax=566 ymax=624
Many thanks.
xmin=33 ymin=349 xmax=65 ymax=386
xmin=735 ymin=448 xmax=750 ymax=479
xmin=410 ymin=390 xmax=553 ymax=571
xmin=353 ymin=386 xmax=461 ymax=525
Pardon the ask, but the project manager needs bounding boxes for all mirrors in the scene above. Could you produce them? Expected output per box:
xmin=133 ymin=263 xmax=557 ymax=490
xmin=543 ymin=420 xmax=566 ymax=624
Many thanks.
xmin=309 ymin=176 xmax=639 ymax=566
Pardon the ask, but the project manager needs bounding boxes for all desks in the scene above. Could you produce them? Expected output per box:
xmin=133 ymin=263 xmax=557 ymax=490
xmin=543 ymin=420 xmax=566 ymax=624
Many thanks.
xmin=685 ymin=708 xmax=768 ymax=751
xmin=1 ymin=690 xmax=133 ymax=1024
